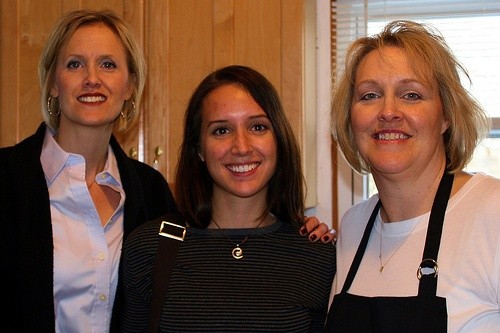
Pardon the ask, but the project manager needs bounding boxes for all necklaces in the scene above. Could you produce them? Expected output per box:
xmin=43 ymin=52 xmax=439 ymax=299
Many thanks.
xmin=379 ymin=207 xmax=432 ymax=273
xmin=86 ymin=153 xmax=105 ymax=189
xmin=210 ymin=211 xmax=264 ymax=260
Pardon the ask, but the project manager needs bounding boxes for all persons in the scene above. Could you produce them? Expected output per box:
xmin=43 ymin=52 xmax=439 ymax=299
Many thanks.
xmin=321 ymin=20 xmax=500 ymax=333
xmin=123 ymin=65 xmax=337 ymax=333
xmin=0 ymin=7 xmax=337 ymax=333
